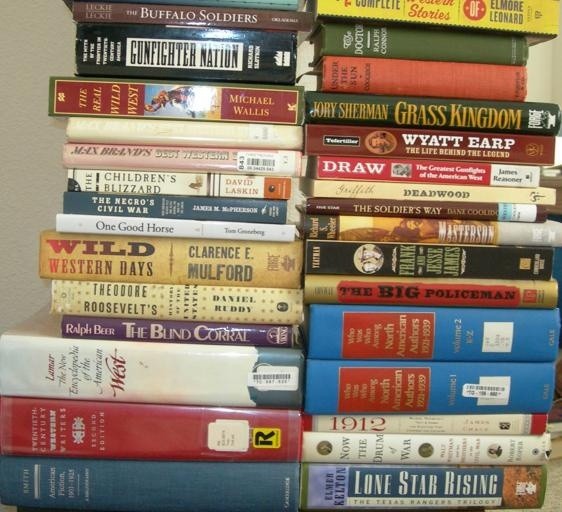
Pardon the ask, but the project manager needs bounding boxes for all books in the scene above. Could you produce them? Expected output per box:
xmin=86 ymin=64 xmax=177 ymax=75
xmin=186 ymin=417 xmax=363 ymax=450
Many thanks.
xmin=2 ymin=0 xmax=562 ymax=511
